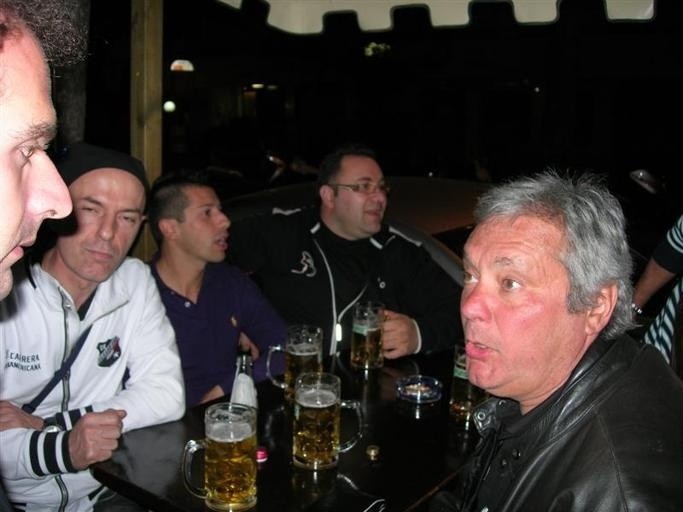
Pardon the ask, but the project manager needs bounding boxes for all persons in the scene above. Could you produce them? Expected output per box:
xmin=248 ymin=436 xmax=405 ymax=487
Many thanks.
xmin=623 ymin=206 xmax=682 ymax=375
xmin=1 ymin=4 xmax=75 ymax=301
xmin=427 ymin=165 xmax=682 ymax=510
xmin=140 ymin=171 xmax=295 ymax=415
xmin=1 ymin=139 xmax=187 ymax=512
xmin=265 ymin=144 xmax=465 ymax=364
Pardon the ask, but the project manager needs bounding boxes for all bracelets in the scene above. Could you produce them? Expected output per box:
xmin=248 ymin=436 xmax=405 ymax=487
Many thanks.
xmin=632 ymin=303 xmax=644 ymax=315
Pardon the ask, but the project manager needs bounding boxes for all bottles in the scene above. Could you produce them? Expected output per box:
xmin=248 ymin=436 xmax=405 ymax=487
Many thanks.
xmin=228 ymin=355 xmax=259 ymax=410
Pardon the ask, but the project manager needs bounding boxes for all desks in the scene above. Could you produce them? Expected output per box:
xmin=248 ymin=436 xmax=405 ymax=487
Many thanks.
xmin=80 ymin=348 xmax=499 ymax=512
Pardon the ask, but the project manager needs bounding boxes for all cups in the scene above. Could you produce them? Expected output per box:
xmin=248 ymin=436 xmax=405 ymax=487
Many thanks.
xmin=348 ymin=300 xmax=387 ymax=370
xmin=264 ymin=324 xmax=324 ymax=400
xmin=446 ymin=339 xmax=492 ymax=416
xmin=289 ymin=372 xmax=367 ymax=473
xmin=179 ymin=399 xmax=259 ymax=511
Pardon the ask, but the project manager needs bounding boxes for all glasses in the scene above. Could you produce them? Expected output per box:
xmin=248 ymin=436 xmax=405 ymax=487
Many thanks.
xmin=327 ymin=183 xmax=390 ymax=196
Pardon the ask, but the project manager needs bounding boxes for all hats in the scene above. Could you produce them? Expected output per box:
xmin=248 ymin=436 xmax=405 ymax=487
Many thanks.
xmin=56 ymin=140 xmax=150 ymax=216
